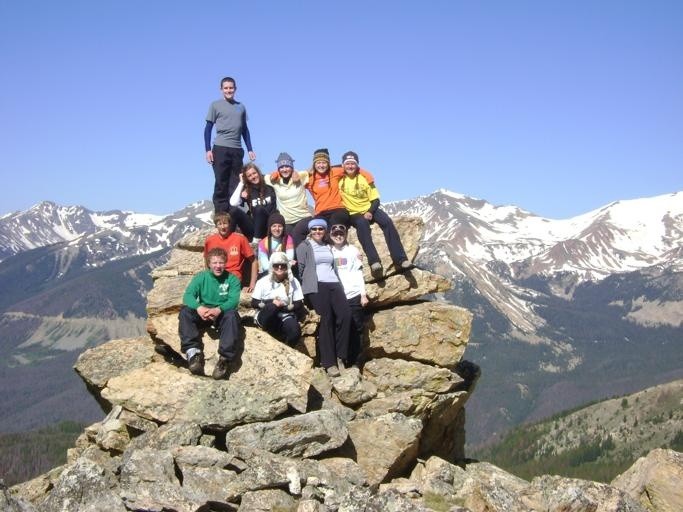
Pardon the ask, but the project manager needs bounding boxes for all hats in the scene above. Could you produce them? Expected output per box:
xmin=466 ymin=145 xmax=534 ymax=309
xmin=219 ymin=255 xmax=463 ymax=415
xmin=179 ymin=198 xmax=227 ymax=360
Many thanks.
xmin=268 ymin=212 xmax=285 ymax=226
xmin=313 ymin=149 xmax=330 ymax=163
xmin=342 ymin=150 xmax=358 ymax=165
xmin=277 ymin=152 xmax=293 ymax=169
xmin=308 ymin=218 xmax=329 ymax=231
xmin=330 ymin=224 xmax=346 ymax=234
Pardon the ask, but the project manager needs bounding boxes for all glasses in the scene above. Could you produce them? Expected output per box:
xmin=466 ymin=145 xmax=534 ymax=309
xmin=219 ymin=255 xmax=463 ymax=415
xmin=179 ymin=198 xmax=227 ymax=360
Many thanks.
xmin=311 ymin=228 xmax=324 ymax=231
xmin=273 ymin=263 xmax=287 ymax=271
xmin=332 ymin=225 xmax=344 ymax=231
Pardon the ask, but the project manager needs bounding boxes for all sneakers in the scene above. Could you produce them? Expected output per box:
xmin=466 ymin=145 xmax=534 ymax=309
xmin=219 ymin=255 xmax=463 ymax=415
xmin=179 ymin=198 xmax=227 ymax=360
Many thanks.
xmin=371 ymin=262 xmax=381 ymax=271
xmin=212 ymin=359 xmax=229 ymax=380
xmin=401 ymin=260 xmax=412 ymax=268
xmin=189 ymin=352 xmax=204 ymax=372
xmin=326 ymin=366 xmax=340 ymax=376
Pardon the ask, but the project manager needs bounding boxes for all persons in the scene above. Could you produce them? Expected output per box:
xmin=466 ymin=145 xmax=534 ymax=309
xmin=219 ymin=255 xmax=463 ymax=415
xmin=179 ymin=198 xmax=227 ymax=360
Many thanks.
xmin=257 ymin=214 xmax=297 ymax=278
xmin=203 ymin=214 xmax=258 ymax=293
xmin=251 ymin=252 xmax=305 ymax=350
xmin=204 ymin=77 xmax=256 ymax=213
xmin=228 ymin=163 xmax=277 ymax=243
xmin=264 ymin=153 xmax=311 ymax=248
xmin=271 ymin=149 xmax=375 ymax=245
xmin=180 ymin=247 xmax=241 ymax=379
xmin=294 ymin=217 xmax=363 ymax=377
xmin=329 ymin=223 xmax=369 ymax=375
xmin=338 ymin=151 xmax=412 ymax=272
